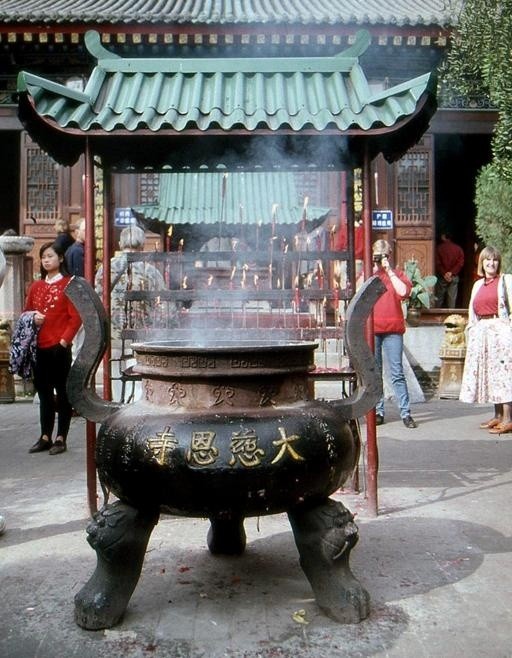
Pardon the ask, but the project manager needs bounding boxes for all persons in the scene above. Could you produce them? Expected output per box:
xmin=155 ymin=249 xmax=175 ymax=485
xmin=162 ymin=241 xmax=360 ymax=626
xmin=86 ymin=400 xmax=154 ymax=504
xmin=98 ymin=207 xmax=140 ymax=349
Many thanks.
xmin=94 ymin=224 xmax=166 ymax=406
xmin=63 ymin=217 xmax=86 ymax=418
xmin=458 ymin=246 xmax=512 ymax=434
xmin=52 ymin=218 xmax=75 ymax=276
xmin=435 ymin=230 xmax=465 ymax=308
xmin=357 ymin=237 xmax=417 ymax=428
xmin=21 ymin=241 xmax=84 ymax=458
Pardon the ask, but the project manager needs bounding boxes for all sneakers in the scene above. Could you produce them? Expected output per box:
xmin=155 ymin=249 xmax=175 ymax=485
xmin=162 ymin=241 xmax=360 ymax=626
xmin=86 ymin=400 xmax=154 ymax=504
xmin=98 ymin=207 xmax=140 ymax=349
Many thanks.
xmin=28 ymin=438 xmax=50 ymax=453
xmin=479 ymin=417 xmax=512 ymax=434
xmin=403 ymin=415 xmax=416 ymax=428
xmin=49 ymin=439 xmax=66 ymax=455
xmin=374 ymin=414 xmax=384 ymax=426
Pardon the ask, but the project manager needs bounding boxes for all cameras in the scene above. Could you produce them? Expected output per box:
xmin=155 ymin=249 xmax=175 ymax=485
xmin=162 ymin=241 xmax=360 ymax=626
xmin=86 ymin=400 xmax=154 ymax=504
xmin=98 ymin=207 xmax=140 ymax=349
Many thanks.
xmin=373 ymin=253 xmax=389 ymax=262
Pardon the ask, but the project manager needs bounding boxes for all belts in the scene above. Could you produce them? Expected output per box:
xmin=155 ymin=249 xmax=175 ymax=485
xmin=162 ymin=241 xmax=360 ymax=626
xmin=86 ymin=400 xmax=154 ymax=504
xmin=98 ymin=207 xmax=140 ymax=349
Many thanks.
xmin=479 ymin=314 xmax=499 ymax=319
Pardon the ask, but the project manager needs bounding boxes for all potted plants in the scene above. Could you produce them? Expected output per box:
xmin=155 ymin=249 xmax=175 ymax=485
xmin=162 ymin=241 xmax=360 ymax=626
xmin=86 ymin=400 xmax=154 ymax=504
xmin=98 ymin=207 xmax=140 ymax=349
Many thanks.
xmin=397 ymin=252 xmax=440 ymax=327
